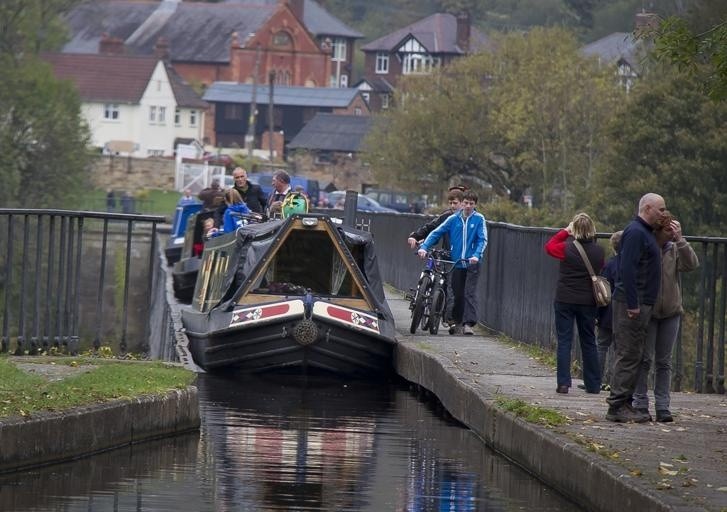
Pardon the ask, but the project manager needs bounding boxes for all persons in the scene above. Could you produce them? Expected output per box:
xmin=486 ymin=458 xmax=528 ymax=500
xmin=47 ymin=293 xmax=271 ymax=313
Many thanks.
xmin=631 ymin=209 xmax=700 ymax=423
xmin=605 ymin=193 xmax=666 ymax=424
xmin=407 ymin=191 xmax=463 ymax=328
xmin=177 ymin=165 xmax=347 ymax=258
xmin=120 ymin=190 xmax=135 ymax=214
xmin=106 ymin=187 xmax=116 ymax=213
xmin=578 ymin=230 xmax=625 ymax=390
xmin=448 ymin=184 xmax=467 ymax=192
xmin=417 ymin=190 xmax=488 ymax=335
xmin=544 ymin=212 xmax=605 ymax=394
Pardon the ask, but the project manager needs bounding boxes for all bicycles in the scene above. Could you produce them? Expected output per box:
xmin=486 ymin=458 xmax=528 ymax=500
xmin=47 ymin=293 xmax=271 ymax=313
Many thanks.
xmin=404 ymin=241 xmax=450 ymax=335
xmin=416 ymin=251 xmax=470 ymax=335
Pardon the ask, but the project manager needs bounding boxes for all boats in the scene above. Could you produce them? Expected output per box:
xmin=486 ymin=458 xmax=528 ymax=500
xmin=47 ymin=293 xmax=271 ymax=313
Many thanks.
xmin=170 ymin=208 xmax=219 ymax=302
xmin=181 ymin=191 xmax=397 ymax=382
xmin=166 ymin=201 xmax=204 ymax=268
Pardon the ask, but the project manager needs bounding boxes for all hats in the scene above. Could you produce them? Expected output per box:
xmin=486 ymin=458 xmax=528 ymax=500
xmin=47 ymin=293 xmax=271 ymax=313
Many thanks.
xmin=213 ymin=173 xmax=257 ymax=188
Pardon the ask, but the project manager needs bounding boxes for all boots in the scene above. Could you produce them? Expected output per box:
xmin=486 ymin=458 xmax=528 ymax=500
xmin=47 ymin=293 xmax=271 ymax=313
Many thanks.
xmin=363 ymin=188 xmax=425 ymax=213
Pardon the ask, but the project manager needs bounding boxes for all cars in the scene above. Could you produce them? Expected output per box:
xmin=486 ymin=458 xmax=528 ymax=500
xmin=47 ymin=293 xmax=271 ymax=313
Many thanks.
xmin=213 ymin=173 xmax=257 ymax=188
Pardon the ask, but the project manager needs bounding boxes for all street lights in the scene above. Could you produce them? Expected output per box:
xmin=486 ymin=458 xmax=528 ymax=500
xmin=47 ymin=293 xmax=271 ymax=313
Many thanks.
xmin=592 ymin=275 xmax=612 ymax=307
xmin=267 ymin=281 xmax=306 ymax=295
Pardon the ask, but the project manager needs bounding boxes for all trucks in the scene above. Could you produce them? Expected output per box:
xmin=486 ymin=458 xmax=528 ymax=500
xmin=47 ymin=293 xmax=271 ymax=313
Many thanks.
xmin=252 ymin=174 xmax=319 ymax=205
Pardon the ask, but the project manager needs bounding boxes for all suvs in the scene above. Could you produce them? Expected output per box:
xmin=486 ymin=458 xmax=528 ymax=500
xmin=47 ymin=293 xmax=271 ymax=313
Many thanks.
xmin=363 ymin=188 xmax=425 ymax=213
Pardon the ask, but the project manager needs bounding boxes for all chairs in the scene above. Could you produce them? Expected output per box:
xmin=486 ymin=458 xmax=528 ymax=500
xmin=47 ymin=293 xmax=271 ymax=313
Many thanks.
xmin=556 ymin=384 xmax=568 ymax=393
xmin=606 ymin=403 xmax=652 ymax=422
xmin=657 ymin=410 xmax=673 ymax=421
xmin=442 ymin=319 xmax=473 ymax=334
xmin=578 ymin=382 xmax=610 ymax=393
